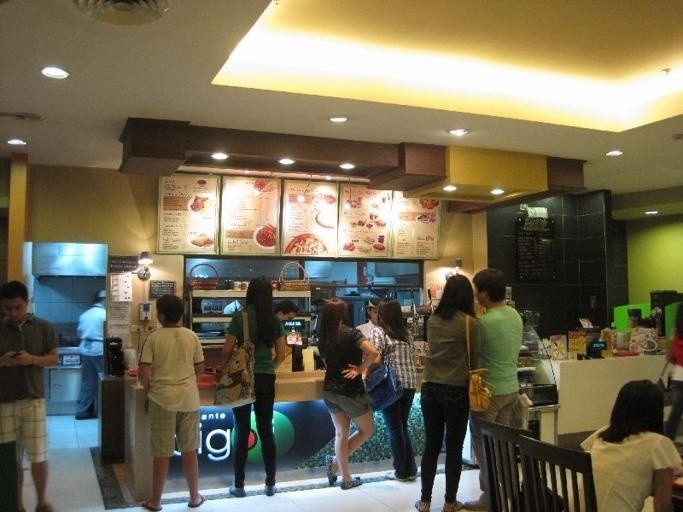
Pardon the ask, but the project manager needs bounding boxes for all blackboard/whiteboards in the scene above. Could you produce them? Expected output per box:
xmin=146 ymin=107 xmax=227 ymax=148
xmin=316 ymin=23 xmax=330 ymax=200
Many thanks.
xmin=150 ymin=281 xmax=175 ymax=299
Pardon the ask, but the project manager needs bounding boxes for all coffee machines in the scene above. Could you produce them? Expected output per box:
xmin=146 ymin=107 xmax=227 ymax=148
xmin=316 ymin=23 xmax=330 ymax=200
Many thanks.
xmin=627 ymin=308 xmax=641 ymax=328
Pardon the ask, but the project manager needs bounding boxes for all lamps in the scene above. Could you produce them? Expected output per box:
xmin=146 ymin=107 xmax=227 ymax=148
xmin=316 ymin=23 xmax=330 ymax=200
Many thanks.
xmin=136 ymin=252 xmax=153 ymax=280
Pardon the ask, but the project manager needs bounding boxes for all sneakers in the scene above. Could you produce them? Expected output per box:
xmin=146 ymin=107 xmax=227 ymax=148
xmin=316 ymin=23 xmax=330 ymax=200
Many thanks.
xmin=443 ymin=501 xmax=463 ymax=512
xmin=325 ymin=455 xmax=337 ymax=485
xmin=385 ymin=470 xmax=416 ymax=482
xmin=229 ymin=487 xmax=245 ymax=497
xmin=340 ymin=477 xmax=363 ymax=489
xmin=265 ymin=486 xmax=277 ymax=496
xmin=464 ymin=501 xmax=488 ymax=511
xmin=415 ymin=500 xmax=430 ymax=512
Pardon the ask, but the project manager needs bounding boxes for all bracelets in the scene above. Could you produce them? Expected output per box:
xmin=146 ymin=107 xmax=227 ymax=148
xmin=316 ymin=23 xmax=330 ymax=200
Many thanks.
xmin=32 ymin=356 xmax=40 ymax=365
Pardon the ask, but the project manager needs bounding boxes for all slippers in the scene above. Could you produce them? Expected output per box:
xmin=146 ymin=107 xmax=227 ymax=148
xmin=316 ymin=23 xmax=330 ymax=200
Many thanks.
xmin=188 ymin=495 xmax=205 ymax=507
xmin=142 ymin=500 xmax=162 ymax=511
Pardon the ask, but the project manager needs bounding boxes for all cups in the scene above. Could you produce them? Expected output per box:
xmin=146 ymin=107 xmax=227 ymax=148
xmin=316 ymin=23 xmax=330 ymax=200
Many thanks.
xmin=219 ymin=277 xmax=251 ymax=292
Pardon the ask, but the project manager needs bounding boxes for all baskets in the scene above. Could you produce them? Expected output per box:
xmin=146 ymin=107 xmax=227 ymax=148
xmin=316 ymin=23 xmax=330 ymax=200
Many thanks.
xmin=189 ymin=264 xmax=218 ymax=290
xmin=279 ymin=262 xmax=310 ymax=291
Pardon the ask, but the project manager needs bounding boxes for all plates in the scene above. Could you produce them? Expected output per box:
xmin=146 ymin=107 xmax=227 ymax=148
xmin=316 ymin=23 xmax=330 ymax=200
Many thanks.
xmin=252 ymin=184 xmax=276 ymax=193
xmin=283 ymin=234 xmax=329 ymax=254
xmin=636 ymin=333 xmax=658 ymax=354
xmin=187 ymin=193 xmax=214 ymax=216
xmin=186 ymin=235 xmax=213 ymax=248
xmin=416 ymin=199 xmax=439 ymax=225
xmin=251 ymin=227 xmax=274 ymax=250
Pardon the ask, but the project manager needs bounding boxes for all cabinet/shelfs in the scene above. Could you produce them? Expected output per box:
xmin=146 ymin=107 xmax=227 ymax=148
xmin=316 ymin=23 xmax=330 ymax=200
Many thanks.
xmin=517 ymin=325 xmax=558 ymax=407
xmin=190 ymin=282 xmax=311 ymax=346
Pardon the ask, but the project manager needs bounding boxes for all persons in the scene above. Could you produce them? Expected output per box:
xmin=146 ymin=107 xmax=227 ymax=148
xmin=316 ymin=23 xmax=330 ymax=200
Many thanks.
xmin=415 ymin=273 xmax=482 ymax=512
xmin=223 ymin=297 xmax=246 ymax=315
xmin=355 ymin=297 xmax=385 ymax=382
xmin=316 ymin=297 xmax=380 ymax=490
xmin=220 ymin=275 xmax=286 ymax=497
xmin=370 ymin=297 xmax=418 ymax=482
xmin=562 ymin=380 xmax=683 ymax=511
xmin=0 ymin=280 xmax=59 ymax=512
xmin=138 ymin=294 xmax=206 ymax=510
xmin=463 ymin=268 xmax=524 ymax=512
xmin=274 ymin=300 xmax=299 ymax=323
xmin=74 ymin=289 xmax=106 ymax=420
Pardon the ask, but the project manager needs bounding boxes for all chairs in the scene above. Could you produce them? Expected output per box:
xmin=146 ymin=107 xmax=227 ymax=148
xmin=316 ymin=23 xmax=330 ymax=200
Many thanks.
xmin=478 ymin=416 xmax=598 ymax=512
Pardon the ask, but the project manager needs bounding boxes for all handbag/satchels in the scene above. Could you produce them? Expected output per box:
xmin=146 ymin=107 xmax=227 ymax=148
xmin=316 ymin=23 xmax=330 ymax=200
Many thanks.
xmin=469 ymin=373 xmax=491 ymax=412
xmin=366 ymin=362 xmax=405 ymax=412
xmin=213 ymin=343 xmax=256 ymax=408
xmin=656 ymin=378 xmax=672 ymax=406
